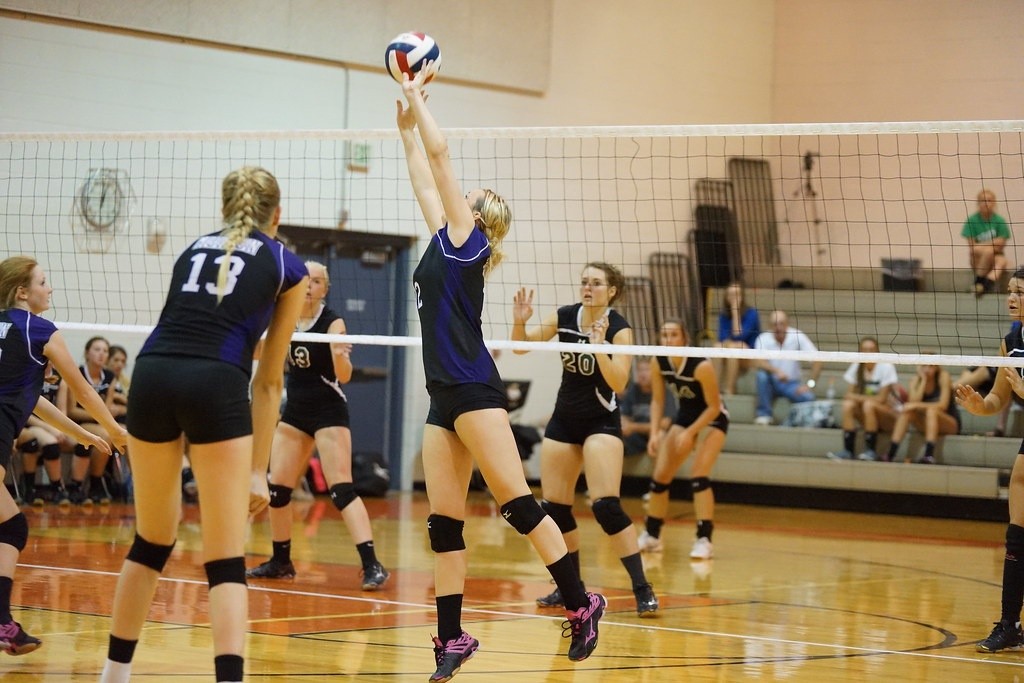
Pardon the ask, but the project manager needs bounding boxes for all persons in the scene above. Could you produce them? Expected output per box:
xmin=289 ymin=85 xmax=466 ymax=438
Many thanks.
xmin=826 ymin=337 xmax=904 ymax=461
xmin=638 ymin=318 xmax=731 ymax=560
xmin=11 ymin=337 xmax=131 ymax=506
xmin=246 ymin=261 xmax=391 ymax=588
xmin=513 ymin=261 xmax=659 ymax=615
xmin=396 ymin=58 xmax=607 ymax=683
xmin=710 ymin=282 xmax=761 ymax=394
xmin=953 ymin=365 xmax=1013 ymax=437
xmin=956 ymin=267 xmax=1024 ymax=653
xmin=752 ymin=310 xmax=823 ymax=424
xmin=100 ymin=167 xmax=312 ymax=683
xmin=619 ymin=357 xmax=678 ymax=500
xmin=961 ymin=190 xmax=1011 ymax=295
xmin=0 ymin=256 xmax=128 ymax=656
xmin=878 ymin=351 xmax=961 ymax=463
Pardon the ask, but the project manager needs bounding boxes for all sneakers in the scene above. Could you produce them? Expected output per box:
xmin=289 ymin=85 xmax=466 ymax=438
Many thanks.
xmin=23 ymin=487 xmax=44 ymax=506
xmin=0 ymin=620 xmax=42 ymax=656
xmin=359 ymin=561 xmax=390 ymax=591
xmin=561 ymin=592 xmax=608 ymax=661
xmin=634 ymin=583 xmax=659 ymax=618
xmin=637 ymin=531 xmax=663 ymax=551
xmin=536 ymin=579 xmax=586 ymax=607
xmin=429 ymin=629 xmax=481 ymax=683
xmin=90 ymin=488 xmax=111 ymax=504
xmin=975 ymin=619 xmax=1024 ymax=653
xmin=69 ymin=481 xmax=93 ymax=504
xmin=51 ymin=487 xmax=69 ymax=505
xmin=246 ymin=556 xmax=296 ymax=580
xmin=690 ymin=537 xmax=713 ymax=558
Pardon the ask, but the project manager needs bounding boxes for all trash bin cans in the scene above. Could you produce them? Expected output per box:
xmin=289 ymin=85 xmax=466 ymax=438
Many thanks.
xmin=882 ymin=259 xmax=918 ymax=291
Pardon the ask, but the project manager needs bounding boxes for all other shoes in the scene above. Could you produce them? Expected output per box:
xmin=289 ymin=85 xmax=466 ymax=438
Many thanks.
xmin=969 ymin=283 xmax=985 ymax=293
xmin=828 ymin=449 xmax=853 ymax=462
xmin=859 ymin=447 xmax=879 ymax=461
xmin=919 ymin=455 xmax=936 ymax=465
xmin=755 ymin=414 xmax=774 ymax=426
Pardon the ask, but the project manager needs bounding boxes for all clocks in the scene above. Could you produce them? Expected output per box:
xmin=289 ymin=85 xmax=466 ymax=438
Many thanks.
xmin=80 ymin=173 xmax=122 ymax=228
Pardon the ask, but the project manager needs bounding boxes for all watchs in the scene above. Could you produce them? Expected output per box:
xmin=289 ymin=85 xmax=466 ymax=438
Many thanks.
xmin=806 ymin=379 xmax=816 ymax=388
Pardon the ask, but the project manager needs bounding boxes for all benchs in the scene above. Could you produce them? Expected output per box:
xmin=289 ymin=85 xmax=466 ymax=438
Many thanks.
xmin=579 ymin=264 xmax=1024 ymax=500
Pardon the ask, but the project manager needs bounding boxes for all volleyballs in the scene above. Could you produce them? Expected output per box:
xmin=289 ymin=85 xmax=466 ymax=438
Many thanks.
xmin=384 ymin=30 xmax=443 ymax=86
